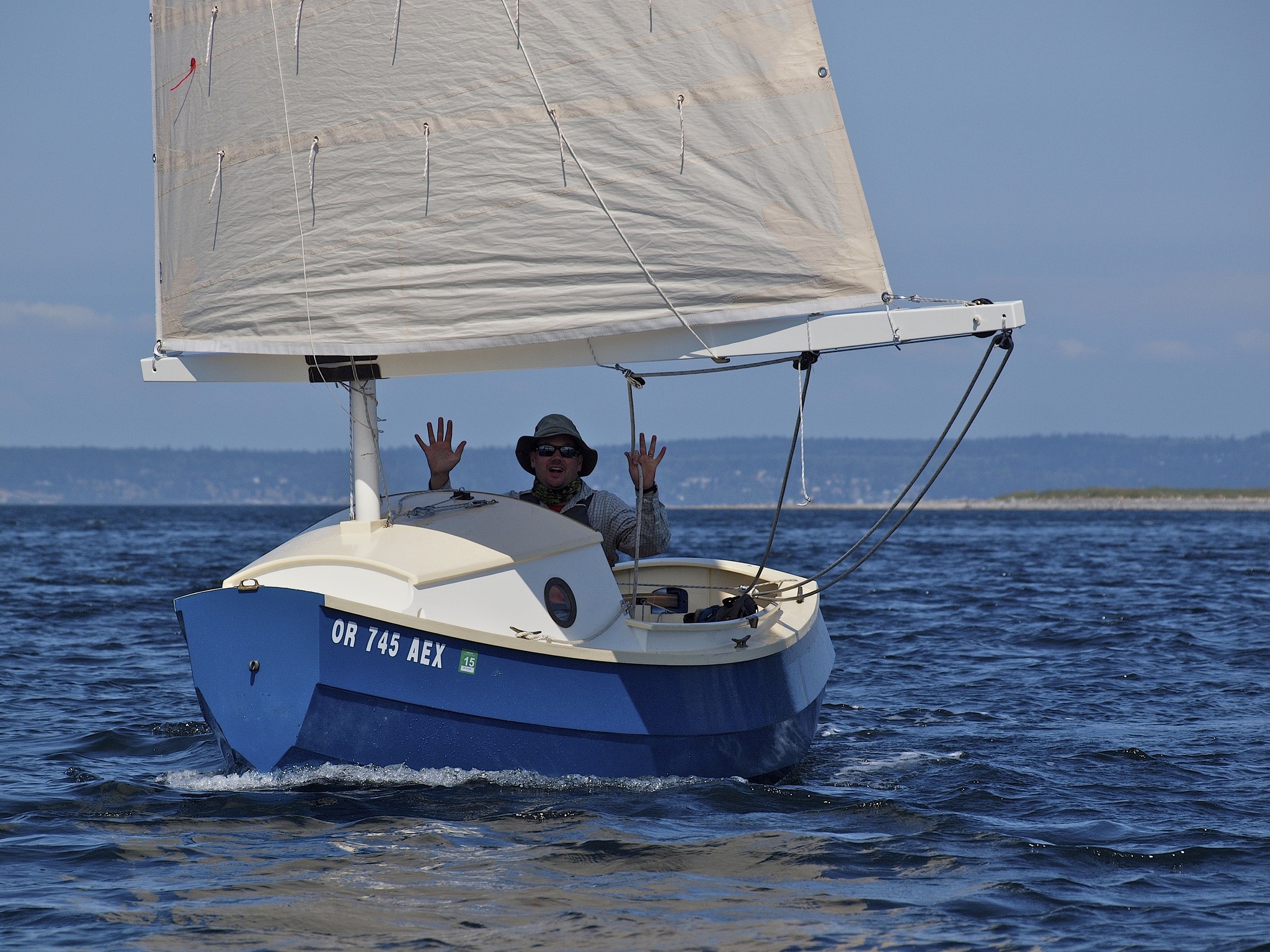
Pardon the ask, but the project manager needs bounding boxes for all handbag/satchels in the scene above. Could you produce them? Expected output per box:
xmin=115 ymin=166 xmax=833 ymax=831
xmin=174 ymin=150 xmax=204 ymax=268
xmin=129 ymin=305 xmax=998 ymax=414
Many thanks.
xmin=682 ymin=593 xmax=758 ymax=623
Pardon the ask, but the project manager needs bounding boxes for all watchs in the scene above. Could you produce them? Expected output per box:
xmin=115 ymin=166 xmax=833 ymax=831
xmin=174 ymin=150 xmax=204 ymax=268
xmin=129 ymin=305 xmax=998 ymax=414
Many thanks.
xmin=636 ymin=481 xmax=658 ymax=494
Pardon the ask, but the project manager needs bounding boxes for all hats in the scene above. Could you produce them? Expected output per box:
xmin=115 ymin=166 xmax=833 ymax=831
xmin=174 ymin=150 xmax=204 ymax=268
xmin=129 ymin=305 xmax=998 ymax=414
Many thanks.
xmin=515 ymin=414 xmax=598 ymax=477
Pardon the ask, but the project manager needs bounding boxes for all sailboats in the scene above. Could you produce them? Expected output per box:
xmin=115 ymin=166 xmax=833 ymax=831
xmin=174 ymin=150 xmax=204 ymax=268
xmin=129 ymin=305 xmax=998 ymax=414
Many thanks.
xmin=141 ymin=0 xmax=1028 ymax=789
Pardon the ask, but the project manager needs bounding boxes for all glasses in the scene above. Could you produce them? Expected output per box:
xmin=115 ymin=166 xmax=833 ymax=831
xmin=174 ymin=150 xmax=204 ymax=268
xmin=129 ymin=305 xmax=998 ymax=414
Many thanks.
xmin=530 ymin=444 xmax=582 ymax=459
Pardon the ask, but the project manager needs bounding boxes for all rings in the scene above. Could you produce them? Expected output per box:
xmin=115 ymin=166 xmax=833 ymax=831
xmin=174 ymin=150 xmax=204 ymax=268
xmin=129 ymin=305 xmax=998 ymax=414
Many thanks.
xmin=648 ymin=454 xmax=654 ymax=458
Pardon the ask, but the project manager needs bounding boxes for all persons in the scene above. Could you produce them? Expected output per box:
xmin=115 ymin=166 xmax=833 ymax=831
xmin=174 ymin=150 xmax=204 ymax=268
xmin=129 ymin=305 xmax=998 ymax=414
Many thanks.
xmin=413 ymin=412 xmax=671 ymax=624
